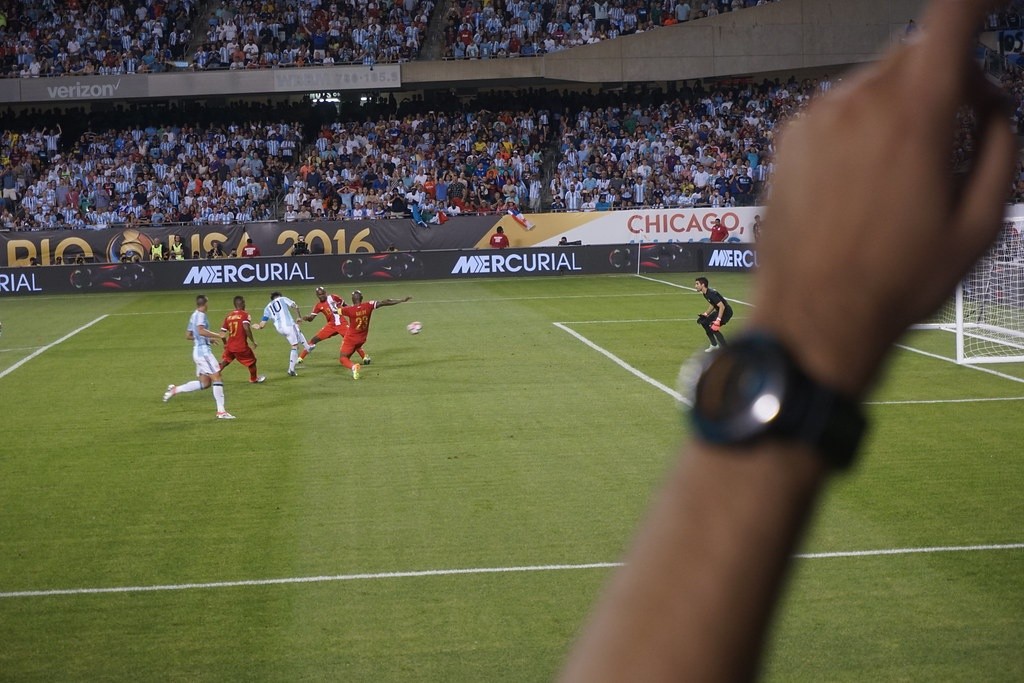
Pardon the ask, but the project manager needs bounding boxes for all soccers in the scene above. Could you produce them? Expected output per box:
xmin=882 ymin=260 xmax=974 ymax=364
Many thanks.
xmin=407 ymin=321 xmax=423 ymax=334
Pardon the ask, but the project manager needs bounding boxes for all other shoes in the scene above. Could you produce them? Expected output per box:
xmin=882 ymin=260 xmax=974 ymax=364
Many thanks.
xmin=297 ymin=358 xmax=304 ymax=365
xmin=364 ymin=354 xmax=371 ymax=364
xmin=289 ymin=369 xmax=297 ymax=376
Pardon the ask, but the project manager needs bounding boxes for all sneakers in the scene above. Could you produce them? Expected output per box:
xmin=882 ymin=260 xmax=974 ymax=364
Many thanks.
xmin=352 ymin=364 xmax=360 ymax=379
xmin=704 ymin=344 xmax=720 ymax=353
xmin=258 ymin=377 xmax=266 ymax=382
xmin=217 ymin=411 xmax=235 ymax=419
xmin=307 ymin=344 xmax=315 ymax=354
xmin=163 ymin=384 xmax=176 ymax=401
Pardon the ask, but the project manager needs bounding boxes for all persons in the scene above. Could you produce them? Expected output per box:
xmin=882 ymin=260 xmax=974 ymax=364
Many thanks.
xmin=293 ymin=234 xmax=310 ymax=255
xmin=148 ymin=235 xmax=183 ymax=261
xmin=251 ymin=291 xmax=316 ymax=376
xmin=217 ymin=296 xmax=266 ymax=383
xmin=334 ymin=290 xmax=412 ymax=379
xmin=710 ymin=219 xmax=729 ymax=242
xmin=119 ymin=253 xmax=141 ymax=263
xmin=242 ymin=239 xmax=260 ymax=257
xmin=490 ymin=226 xmax=509 ymax=249
xmin=194 ymin=242 xmax=237 ymax=258
xmin=560 ymin=0 xmax=1017 ymax=683
xmin=0 ymin=0 xmax=773 ymax=78
xmin=561 ymin=237 xmax=567 ymax=244
xmin=0 ymin=74 xmax=831 ymax=232
xmin=695 ymin=277 xmax=733 ymax=353
xmin=753 ymin=214 xmax=763 ymax=242
xmin=162 ymin=294 xmax=236 ymax=419
xmin=29 ymin=256 xmax=85 ymax=266
xmin=297 ymin=287 xmax=371 ymax=365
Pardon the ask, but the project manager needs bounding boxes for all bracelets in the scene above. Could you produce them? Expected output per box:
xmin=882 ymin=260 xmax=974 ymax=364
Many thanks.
xmin=717 ymin=317 xmax=721 ymax=321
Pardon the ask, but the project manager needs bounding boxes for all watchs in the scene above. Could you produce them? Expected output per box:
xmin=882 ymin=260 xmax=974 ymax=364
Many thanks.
xmin=685 ymin=330 xmax=867 ymax=474
